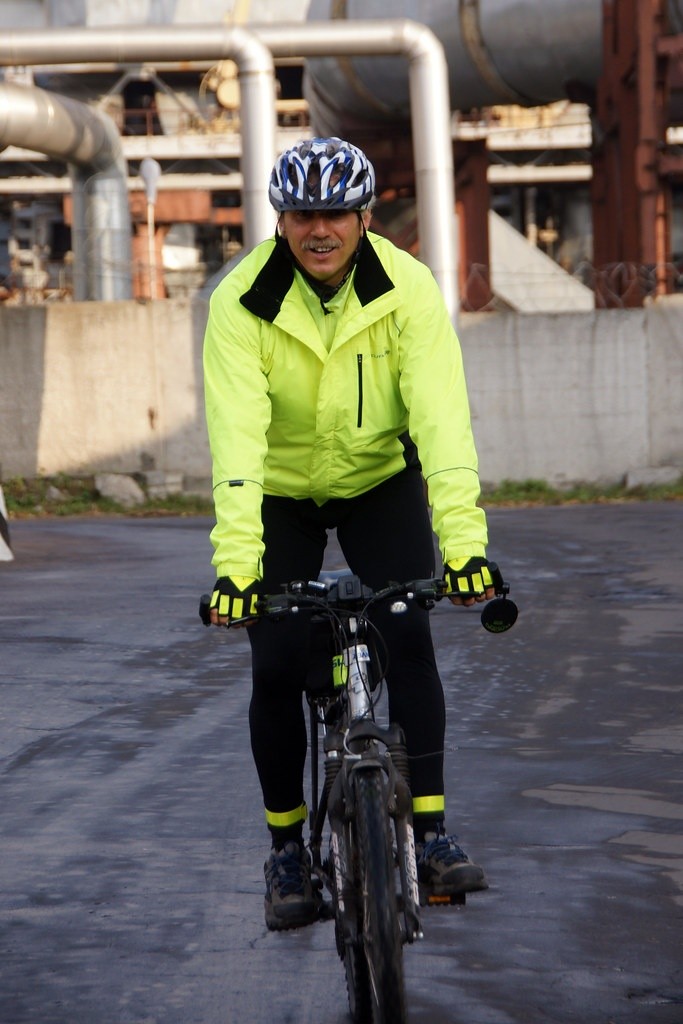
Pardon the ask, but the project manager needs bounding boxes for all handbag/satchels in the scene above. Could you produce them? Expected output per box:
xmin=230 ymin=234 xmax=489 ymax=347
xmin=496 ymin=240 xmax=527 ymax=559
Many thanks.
xmin=306 ymin=620 xmax=380 ymax=699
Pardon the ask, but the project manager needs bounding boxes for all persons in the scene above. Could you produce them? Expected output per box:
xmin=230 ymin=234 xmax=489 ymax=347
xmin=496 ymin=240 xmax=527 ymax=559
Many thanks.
xmin=202 ymin=136 xmax=496 ymax=919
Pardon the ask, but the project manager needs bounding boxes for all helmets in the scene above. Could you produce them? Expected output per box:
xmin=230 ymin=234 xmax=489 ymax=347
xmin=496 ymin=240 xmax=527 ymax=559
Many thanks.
xmin=269 ymin=137 xmax=377 ymax=212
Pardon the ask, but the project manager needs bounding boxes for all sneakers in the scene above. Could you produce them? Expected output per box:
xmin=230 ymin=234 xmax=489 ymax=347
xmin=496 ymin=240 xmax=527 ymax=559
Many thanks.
xmin=415 ymin=831 xmax=489 ymax=896
xmin=264 ymin=838 xmax=322 ymax=932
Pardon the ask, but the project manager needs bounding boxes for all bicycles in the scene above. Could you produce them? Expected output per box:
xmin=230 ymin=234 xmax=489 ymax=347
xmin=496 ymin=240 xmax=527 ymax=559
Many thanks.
xmin=198 ymin=561 xmax=521 ymax=1024
